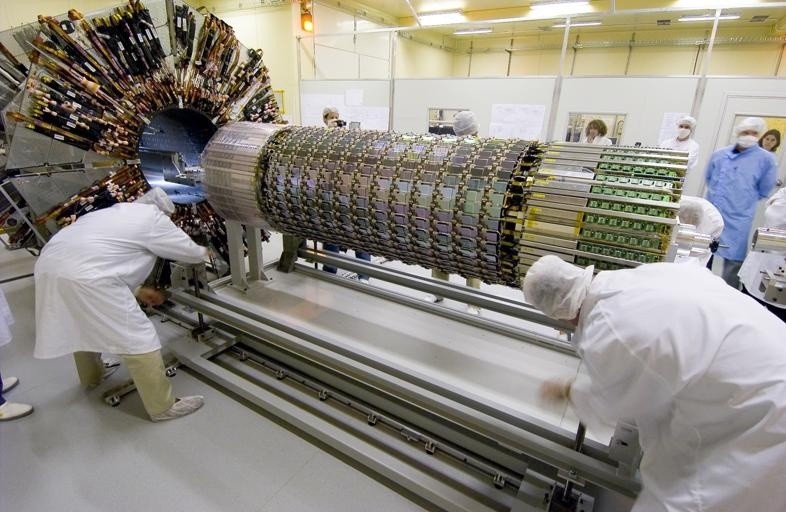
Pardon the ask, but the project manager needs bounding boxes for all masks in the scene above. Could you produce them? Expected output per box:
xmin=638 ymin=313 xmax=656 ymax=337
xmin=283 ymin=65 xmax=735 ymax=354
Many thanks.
xmin=737 ymin=135 xmax=758 ymax=148
xmin=328 ymin=119 xmax=339 ymax=127
xmin=678 ymin=128 xmax=691 ymax=138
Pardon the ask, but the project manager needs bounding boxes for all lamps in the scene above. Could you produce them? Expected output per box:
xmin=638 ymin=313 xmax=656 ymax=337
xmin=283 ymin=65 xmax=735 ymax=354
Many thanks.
xmin=676 ymin=13 xmax=743 ymax=23
xmin=452 ymin=21 xmax=605 ymax=36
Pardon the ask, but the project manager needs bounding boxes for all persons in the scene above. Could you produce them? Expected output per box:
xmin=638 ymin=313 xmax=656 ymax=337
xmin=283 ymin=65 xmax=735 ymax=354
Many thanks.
xmin=582 ymin=119 xmax=614 ymax=145
xmin=759 ymin=129 xmax=782 ymax=153
xmin=0 ymin=287 xmax=33 ymax=421
xmin=452 ymin=110 xmax=481 ymax=138
xmin=522 ymin=253 xmax=786 ymax=511
xmin=321 ymin=108 xmax=346 ymax=129
xmin=677 ymin=195 xmax=725 ymax=272
xmin=660 ymin=116 xmax=701 ymax=172
xmin=31 ymin=186 xmax=221 ymax=421
xmin=320 ymin=242 xmax=370 ymax=284
xmin=707 ymin=114 xmax=779 ymax=288
xmin=736 ymin=184 xmax=786 ymax=322
xmin=430 ymin=267 xmax=481 ymax=317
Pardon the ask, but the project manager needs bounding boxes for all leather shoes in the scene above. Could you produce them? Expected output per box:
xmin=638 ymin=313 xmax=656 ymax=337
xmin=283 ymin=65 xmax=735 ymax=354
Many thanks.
xmin=0 ymin=401 xmax=34 ymax=421
xmin=2 ymin=376 xmax=19 ymax=392
